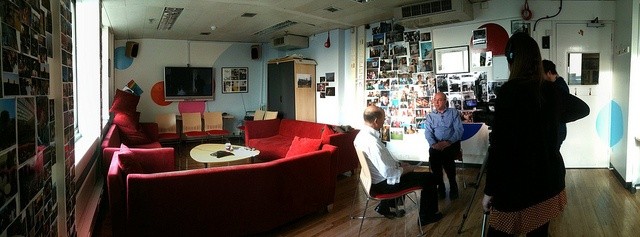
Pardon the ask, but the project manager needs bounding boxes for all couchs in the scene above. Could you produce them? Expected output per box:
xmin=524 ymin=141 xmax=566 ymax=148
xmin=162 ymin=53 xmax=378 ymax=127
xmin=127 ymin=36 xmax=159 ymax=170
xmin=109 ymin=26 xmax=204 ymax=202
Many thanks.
xmin=243 ymin=118 xmax=360 ymax=176
xmin=106 ymin=146 xmax=175 ymax=226
xmin=126 ymin=144 xmax=338 ymax=237
xmin=102 ymin=120 xmax=162 ymax=182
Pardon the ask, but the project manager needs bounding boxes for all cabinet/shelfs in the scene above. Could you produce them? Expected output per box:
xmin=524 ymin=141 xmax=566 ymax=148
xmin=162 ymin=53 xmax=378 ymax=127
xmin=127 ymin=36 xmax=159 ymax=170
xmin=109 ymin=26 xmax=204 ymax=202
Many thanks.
xmin=267 ymin=60 xmax=317 ymax=123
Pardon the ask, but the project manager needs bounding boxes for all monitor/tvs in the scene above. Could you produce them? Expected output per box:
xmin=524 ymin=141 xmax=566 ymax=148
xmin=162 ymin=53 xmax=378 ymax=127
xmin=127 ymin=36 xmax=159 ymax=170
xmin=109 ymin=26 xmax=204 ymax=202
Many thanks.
xmin=163 ymin=66 xmax=215 ymax=102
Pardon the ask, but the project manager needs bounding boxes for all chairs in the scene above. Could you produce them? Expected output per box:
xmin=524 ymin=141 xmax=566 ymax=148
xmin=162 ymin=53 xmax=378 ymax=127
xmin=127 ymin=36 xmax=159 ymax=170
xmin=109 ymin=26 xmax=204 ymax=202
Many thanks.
xmin=154 ymin=113 xmax=180 ymax=156
xmin=349 ymin=141 xmax=425 ymax=237
xmin=203 ymin=110 xmax=231 ymax=144
xmin=181 ymin=112 xmax=209 ymax=141
xmin=252 ymin=110 xmax=265 ymax=121
xmin=264 ymin=110 xmax=279 ymax=121
xmin=428 ymin=138 xmax=467 ymax=190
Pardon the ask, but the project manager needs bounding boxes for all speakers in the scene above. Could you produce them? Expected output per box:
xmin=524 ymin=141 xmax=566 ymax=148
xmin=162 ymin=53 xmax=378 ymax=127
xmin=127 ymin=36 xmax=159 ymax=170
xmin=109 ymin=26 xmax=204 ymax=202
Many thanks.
xmin=250 ymin=45 xmax=260 ymax=60
xmin=125 ymin=41 xmax=139 ymax=58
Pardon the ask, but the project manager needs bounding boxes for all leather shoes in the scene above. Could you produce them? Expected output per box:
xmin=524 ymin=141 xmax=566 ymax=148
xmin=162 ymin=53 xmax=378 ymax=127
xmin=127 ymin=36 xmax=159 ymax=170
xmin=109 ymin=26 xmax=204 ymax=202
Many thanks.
xmin=418 ymin=212 xmax=442 ymax=226
xmin=374 ymin=202 xmax=395 ymax=219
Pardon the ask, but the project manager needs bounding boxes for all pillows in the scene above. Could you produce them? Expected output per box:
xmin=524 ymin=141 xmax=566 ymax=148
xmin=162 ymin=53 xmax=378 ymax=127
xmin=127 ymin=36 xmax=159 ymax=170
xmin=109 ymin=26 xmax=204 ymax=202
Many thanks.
xmin=116 ymin=142 xmax=142 ymax=175
xmin=109 ymin=88 xmax=140 ymax=113
xmin=284 ymin=135 xmax=323 ymax=162
xmin=320 ymin=124 xmax=338 ymax=145
xmin=112 ymin=111 xmax=141 ymax=128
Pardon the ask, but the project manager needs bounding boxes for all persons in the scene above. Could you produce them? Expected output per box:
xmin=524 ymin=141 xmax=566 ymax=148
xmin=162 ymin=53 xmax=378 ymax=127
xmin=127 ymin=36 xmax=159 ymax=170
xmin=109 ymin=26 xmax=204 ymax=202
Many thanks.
xmin=382 ymin=64 xmax=386 ymax=72
xmin=380 ymin=93 xmax=388 ymax=106
xmin=378 ymin=81 xmax=384 ymax=89
xmin=421 ymin=63 xmax=427 ymax=72
xmin=405 ymin=125 xmax=415 ymax=134
xmin=424 ymin=93 xmax=464 ymax=200
xmin=37 ymin=108 xmax=51 ymax=145
xmin=460 ymin=32 xmax=589 ymax=236
xmin=416 ymin=75 xmax=425 ymax=85
xmin=542 ymin=60 xmax=570 ymax=95
xmin=366 ymin=81 xmax=374 ymax=89
xmin=353 ymin=107 xmax=441 ymax=227
xmin=402 ymin=58 xmax=407 ymax=64
xmin=410 ymin=59 xmax=418 ymax=64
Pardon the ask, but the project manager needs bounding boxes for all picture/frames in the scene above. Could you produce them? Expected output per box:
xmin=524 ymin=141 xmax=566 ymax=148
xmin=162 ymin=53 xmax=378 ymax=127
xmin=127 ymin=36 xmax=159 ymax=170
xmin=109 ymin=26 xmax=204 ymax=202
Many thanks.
xmin=221 ymin=67 xmax=249 ymax=93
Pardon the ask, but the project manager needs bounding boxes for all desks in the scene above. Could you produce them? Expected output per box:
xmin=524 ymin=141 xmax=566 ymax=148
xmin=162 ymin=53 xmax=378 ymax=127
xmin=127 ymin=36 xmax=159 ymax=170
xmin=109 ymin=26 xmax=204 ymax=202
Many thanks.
xmin=175 ymin=112 xmax=235 ymax=122
